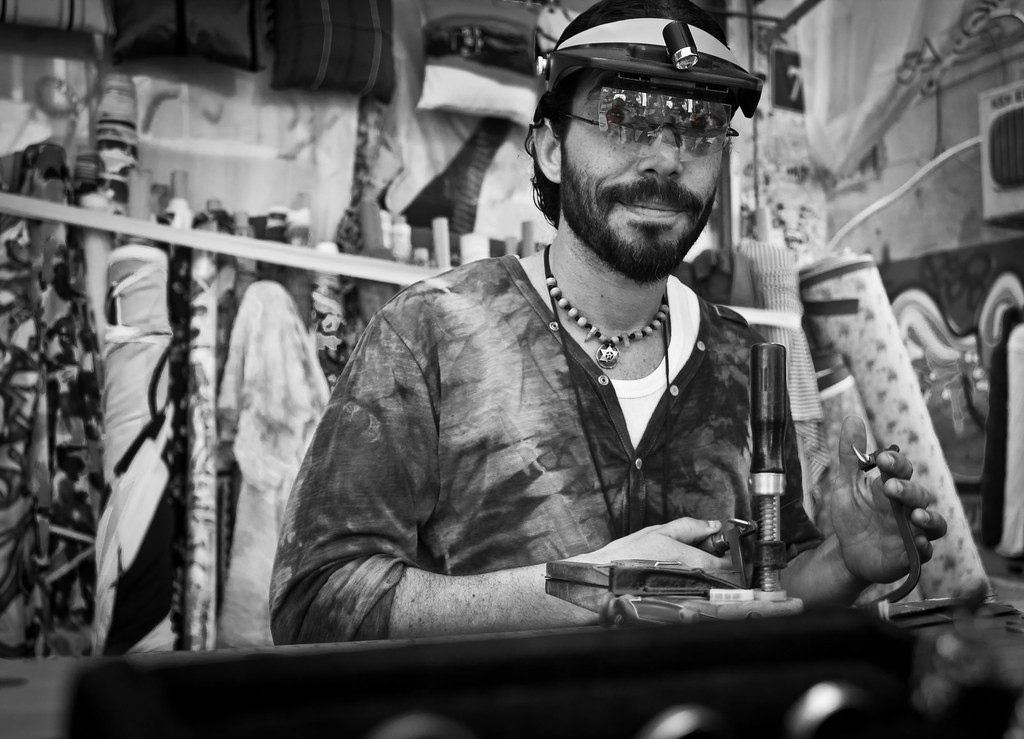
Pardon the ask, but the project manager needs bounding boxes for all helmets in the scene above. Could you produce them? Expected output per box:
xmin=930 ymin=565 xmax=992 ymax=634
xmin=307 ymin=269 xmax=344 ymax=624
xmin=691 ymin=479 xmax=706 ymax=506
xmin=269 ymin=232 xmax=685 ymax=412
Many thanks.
xmin=534 ymin=20 xmax=764 ymax=122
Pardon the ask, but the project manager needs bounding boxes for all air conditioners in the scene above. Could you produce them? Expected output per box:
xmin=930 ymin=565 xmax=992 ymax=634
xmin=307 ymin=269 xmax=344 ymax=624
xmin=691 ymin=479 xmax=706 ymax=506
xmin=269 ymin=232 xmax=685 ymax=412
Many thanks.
xmin=978 ymin=80 xmax=1024 ymax=231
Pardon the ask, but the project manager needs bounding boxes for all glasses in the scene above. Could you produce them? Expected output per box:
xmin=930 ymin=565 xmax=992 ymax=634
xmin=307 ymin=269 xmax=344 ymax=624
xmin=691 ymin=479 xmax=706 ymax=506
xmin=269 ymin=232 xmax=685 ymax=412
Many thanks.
xmin=561 ymin=89 xmax=737 ymax=154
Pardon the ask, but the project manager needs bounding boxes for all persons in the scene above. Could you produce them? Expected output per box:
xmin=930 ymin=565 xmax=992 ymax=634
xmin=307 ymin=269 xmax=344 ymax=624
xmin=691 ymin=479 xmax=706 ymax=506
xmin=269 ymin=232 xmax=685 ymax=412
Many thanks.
xmin=268 ymin=0 xmax=947 ymax=645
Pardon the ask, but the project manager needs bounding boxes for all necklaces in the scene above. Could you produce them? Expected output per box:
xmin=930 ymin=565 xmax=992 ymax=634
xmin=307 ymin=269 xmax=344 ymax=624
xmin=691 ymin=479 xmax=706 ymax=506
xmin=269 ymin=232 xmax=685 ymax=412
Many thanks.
xmin=541 ymin=240 xmax=670 ymax=370
